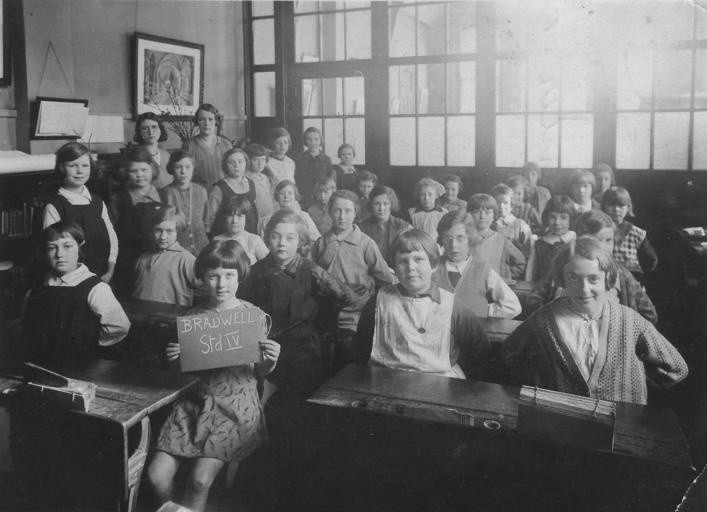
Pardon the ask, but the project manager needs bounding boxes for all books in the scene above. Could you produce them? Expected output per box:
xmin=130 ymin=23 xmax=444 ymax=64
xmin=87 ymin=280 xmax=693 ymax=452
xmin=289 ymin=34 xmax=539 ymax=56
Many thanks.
xmin=1 ymin=196 xmax=47 ymax=236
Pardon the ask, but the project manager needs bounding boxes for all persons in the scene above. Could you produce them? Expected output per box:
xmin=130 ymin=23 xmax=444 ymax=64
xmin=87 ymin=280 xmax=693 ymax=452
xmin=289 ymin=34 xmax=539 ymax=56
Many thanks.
xmin=22 ymin=104 xmax=688 ymax=512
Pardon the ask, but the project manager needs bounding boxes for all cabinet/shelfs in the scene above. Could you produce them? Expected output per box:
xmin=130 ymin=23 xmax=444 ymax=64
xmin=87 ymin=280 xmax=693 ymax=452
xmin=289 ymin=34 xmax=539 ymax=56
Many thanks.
xmin=0 ymin=168 xmax=60 ymax=330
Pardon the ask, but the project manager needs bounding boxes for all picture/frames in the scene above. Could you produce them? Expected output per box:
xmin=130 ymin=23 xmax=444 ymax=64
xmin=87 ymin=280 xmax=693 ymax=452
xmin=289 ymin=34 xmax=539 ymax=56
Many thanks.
xmin=132 ymin=31 xmax=205 ymax=121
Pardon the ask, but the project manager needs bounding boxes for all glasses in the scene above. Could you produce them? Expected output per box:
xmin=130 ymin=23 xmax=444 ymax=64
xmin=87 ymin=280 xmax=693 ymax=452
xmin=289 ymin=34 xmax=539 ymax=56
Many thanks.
xmin=439 ymin=234 xmax=468 ymax=241
